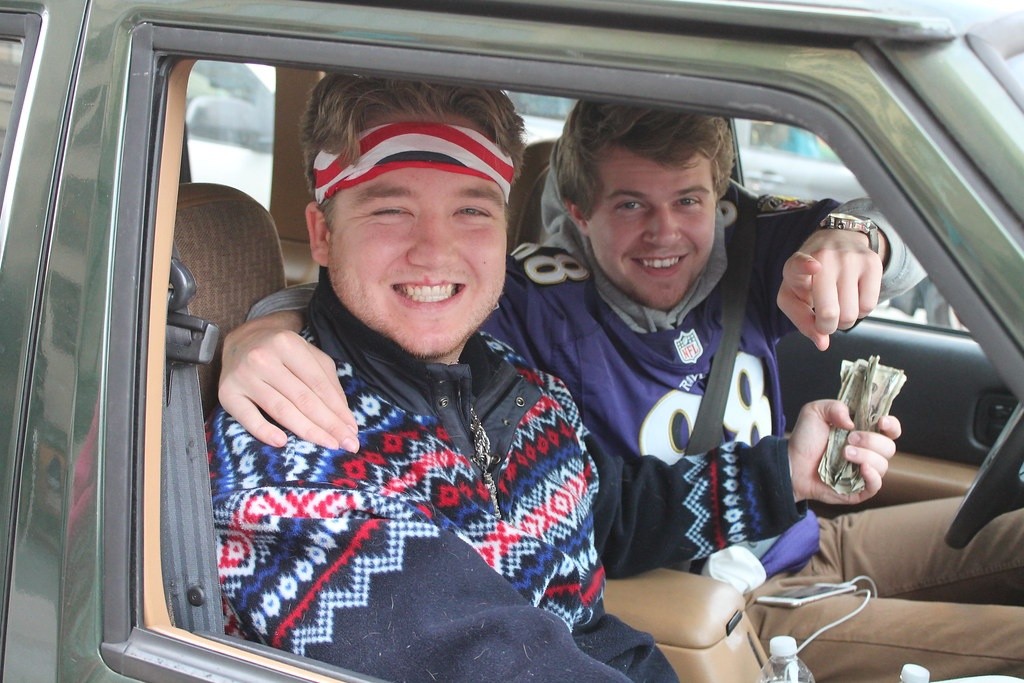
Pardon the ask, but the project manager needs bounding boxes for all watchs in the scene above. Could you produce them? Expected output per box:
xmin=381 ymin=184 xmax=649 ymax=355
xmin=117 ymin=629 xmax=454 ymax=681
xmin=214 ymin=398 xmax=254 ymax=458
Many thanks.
xmin=819 ymin=212 xmax=879 ymax=255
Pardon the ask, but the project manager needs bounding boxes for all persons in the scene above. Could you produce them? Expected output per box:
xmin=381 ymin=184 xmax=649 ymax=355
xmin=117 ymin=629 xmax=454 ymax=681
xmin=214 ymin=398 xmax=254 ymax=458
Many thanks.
xmin=218 ymin=100 xmax=1024 ymax=683
xmin=204 ymin=72 xmax=902 ymax=683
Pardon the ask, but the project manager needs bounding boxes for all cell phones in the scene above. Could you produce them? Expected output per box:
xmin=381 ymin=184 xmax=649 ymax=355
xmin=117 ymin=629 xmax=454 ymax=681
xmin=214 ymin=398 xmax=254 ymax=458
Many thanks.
xmin=755 ymin=584 xmax=857 ymax=609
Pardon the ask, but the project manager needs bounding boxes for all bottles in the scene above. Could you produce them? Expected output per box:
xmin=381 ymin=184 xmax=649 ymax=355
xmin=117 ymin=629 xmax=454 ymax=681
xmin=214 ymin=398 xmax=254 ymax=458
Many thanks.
xmin=898 ymin=664 xmax=931 ymax=683
xmin=757 ymin=636 xmax=816 ymax=683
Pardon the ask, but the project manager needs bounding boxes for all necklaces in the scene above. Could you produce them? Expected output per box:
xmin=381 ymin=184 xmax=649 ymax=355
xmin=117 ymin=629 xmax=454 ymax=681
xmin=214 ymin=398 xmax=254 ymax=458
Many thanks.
xmin=470 ymin=407 xmax=502 ymax=522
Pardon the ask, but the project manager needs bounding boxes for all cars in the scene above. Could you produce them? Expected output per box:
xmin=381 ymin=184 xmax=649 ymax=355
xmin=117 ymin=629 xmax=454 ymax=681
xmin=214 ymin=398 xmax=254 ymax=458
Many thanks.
xmin=182 ymin=52 xmax=284 ymax=214
xmin=731 ymin=114 xmax=971 ymax=331
xmin=0 ymin=0 xmax=1024 ymax=680
